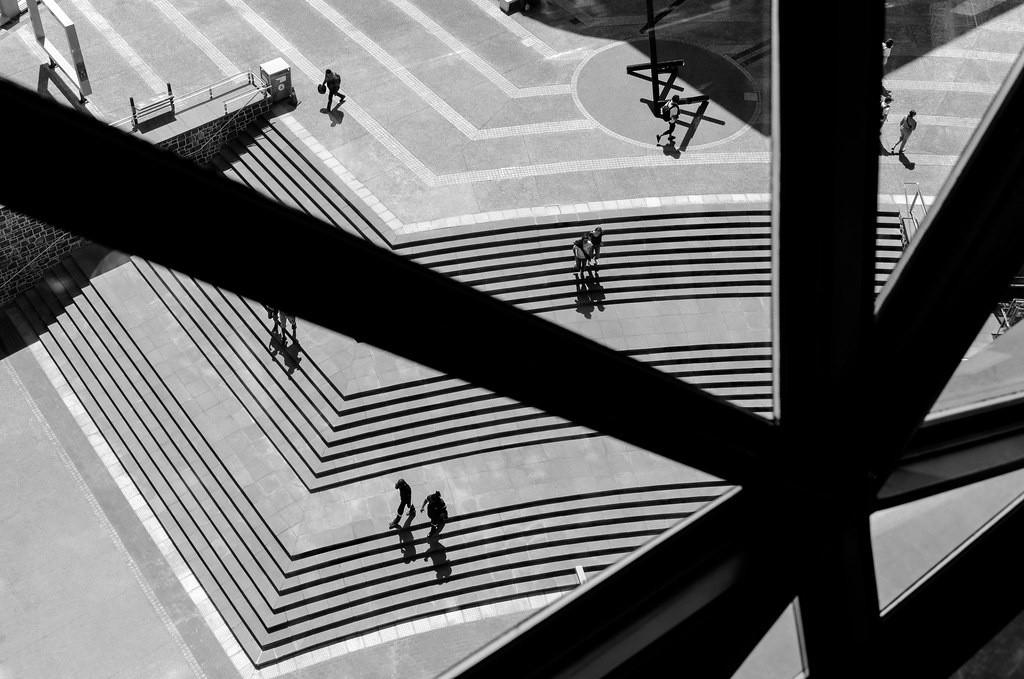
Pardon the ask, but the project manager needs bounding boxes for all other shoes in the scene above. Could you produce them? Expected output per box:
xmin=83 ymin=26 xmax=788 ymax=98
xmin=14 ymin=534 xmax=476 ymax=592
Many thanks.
xmin=587 ymin=261 xmax=591 ymax=267
xmin=406 ymin=504 xmax=416 ymax=515
xmin=273 ymin=325 xmax=278 ymax=331
xmin=389 ymin=515 xmax=401 ymax=528
xmin=573 ymin=263 xmax=576 ymax=271
xmin=581 ymin=272 xmax=584 ymax=276
xmin=593 ymin=256 xmax=597 ymax=265
xmin=281 ymin=323 xmax=296 ymax=334
xmin=892 ymin=148 xmax=894 ymax=154
xmin=427 ymin=528 xmax=435 ymax=538
xmin=668 ymin=136 xmax=675 ymax=140
xmin=656 ymin=135 xmax=660 ymax=143
xmin=899 ymin=149 xmax=905 ymax=153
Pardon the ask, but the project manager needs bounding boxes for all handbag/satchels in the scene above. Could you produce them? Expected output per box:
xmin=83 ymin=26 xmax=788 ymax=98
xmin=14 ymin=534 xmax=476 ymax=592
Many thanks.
xmin=430 ymin=532 xmax=439 ymax=544
xmin=317 ymin=84 xmax=326 ymax=94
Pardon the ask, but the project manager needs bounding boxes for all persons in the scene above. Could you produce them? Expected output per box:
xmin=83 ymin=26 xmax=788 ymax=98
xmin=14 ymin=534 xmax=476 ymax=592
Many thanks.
xmin=882 ymin=38 xmax=894 ymax=71
xmin=572 ymin=233 xmax=593 ymax=273
xmin=588 ymin=226 xmax=604 ymax=266
xmin=388 ymin=478 xmax=416 ymax=527
xmin=891 ymin=109 xmax=918 ymax=153
xmin=321 ymin=68 xmax=346 ymax=112
xmin=656 ymin=94 xmax=681 ymax=144
xmin=278 ymin=310 xmax=297 ymax=347
xmin=420 ymin=490 xmax=448 ymax=538
xmin=880 ymin=96 xmax=894 ymax=135
xmin=263 ymin=303 xmax=279 ymax=332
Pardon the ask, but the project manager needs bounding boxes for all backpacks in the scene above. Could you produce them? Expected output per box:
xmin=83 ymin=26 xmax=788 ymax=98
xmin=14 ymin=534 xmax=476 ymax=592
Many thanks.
xmin=333 ymin=73 xmax=341 ymax=85
xmin=663 ymin=102 xmax=677 ymax=122
xmin=880 ymin=105 xmax=890 ymax=118
